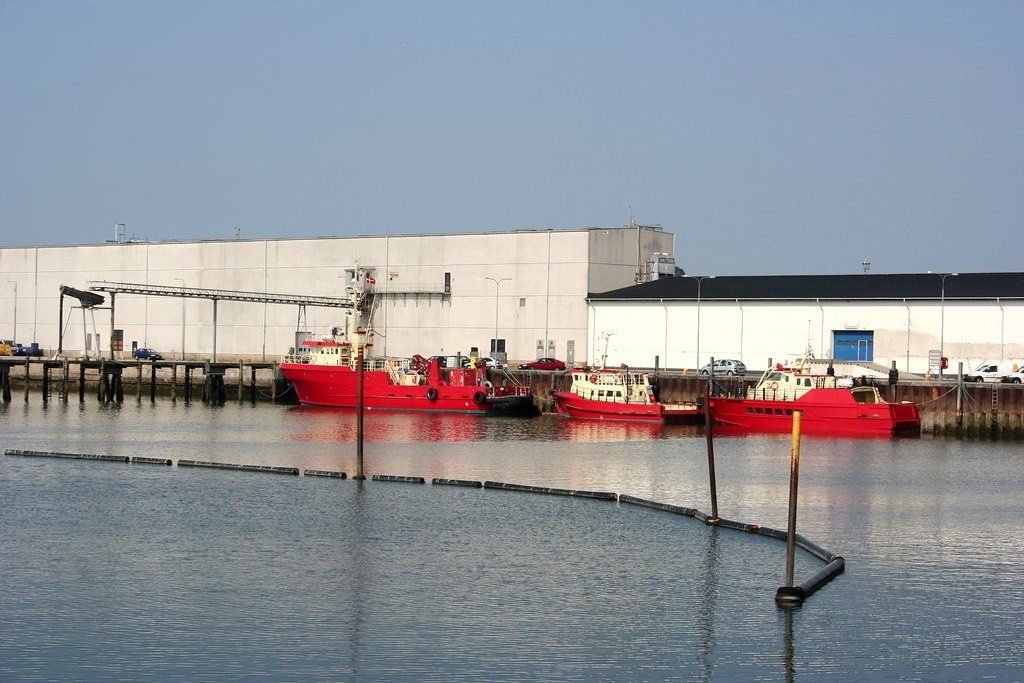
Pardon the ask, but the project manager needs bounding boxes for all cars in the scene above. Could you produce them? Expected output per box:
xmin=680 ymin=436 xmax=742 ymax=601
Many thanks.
xmin=428 ymin=355 xmax=471 ymax=369
xmin=517 ymin=357 xmax=566 ymax=371
xmin=0 ymin=339 xmax=19 ymax=355
xmin=463 ymin=357 xmax=508 ymax=369
xmin=700 ymin=359 xmax=747 ymax=376
xmin=133 ymin=348 xmax=161 ymax=360
xmin=1001 ymin=366 xmax=1024 ymax=384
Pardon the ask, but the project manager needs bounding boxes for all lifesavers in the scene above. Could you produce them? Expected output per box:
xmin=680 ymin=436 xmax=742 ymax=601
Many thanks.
xmin=696 ymin=397 xmax=704 ymax=409
xmin=771 ymin=382 xmax=779 ymax=391
xmin=426 ymin=388 xmax=438 ymax=401
xmin=589 ymin=376 xmax=597 ymax=383
xmin=474 ymin=392 xmax=486 ymax=404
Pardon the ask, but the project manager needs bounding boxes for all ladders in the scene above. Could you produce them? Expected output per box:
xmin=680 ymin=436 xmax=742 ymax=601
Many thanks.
xmin=991 ymin=384 xmax=998 ymax=409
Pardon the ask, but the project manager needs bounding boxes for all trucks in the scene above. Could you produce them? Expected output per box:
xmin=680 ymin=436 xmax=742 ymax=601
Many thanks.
xmin=963 ymin=363 xmax=1018 ymax=383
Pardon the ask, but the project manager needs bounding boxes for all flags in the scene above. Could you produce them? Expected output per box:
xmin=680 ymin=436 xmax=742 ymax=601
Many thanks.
xmin=366 ymin=275 xmax=375 ymax=284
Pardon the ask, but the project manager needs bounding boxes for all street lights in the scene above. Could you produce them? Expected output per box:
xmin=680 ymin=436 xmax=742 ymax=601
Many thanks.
xmin=681 ymin=273 xmax=715 ymax=375
xmin=928 ymin=270 xmax=959 ymax=383
xmin=485 ymin=276 xmax=513 ymax=368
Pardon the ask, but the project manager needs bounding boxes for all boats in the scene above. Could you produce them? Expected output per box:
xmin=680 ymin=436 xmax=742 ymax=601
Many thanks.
xmin=699 ymin=320 xmax=922 ymax=439
xmin=548 ymin=332 xmax=704 ymax=425
xmin=280 ymin=327 xmax=534 ymax=415
